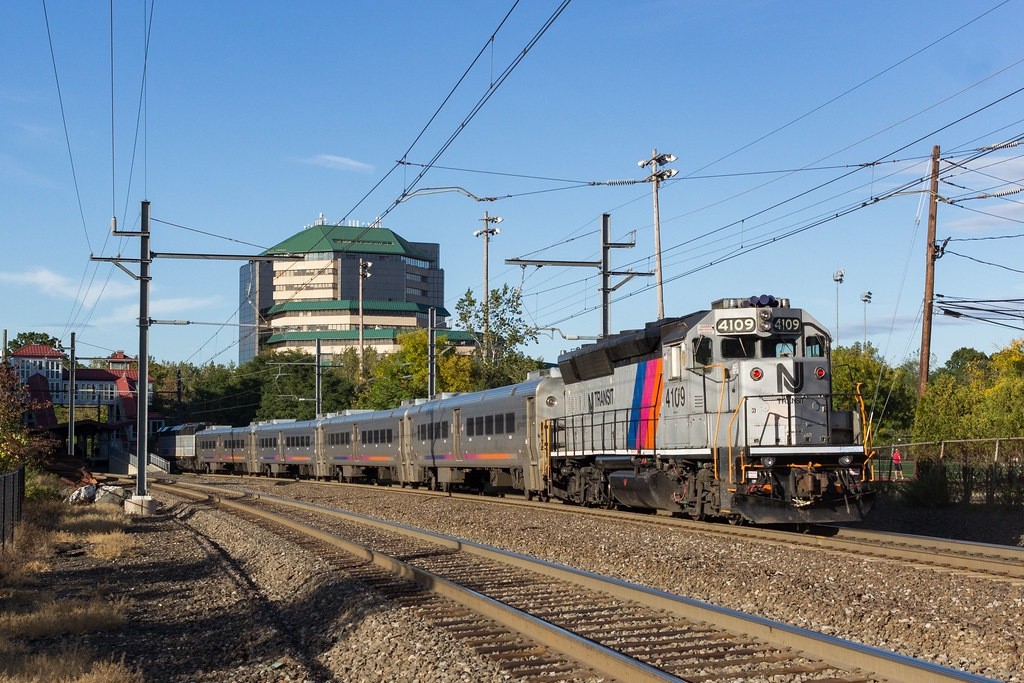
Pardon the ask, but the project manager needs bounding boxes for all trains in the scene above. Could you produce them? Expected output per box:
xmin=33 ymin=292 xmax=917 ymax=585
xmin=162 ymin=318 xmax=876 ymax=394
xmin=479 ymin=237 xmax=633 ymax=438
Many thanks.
xmin=153 ymin=293 xmax=890 ymax=536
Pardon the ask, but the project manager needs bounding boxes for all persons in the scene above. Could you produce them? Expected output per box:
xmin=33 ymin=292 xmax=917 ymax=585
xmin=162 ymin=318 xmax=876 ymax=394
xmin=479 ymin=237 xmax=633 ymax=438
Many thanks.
xmin=893 ymin=448 xmax=906 ymax=480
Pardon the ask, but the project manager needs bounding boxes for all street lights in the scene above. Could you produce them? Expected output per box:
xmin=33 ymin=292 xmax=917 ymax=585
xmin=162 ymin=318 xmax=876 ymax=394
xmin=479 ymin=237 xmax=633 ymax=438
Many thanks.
xmin=831 ymin=268 xmax=845 ymax=346
xmin=474 ymin=210 xmax=504 ymax=364
xmin=860 ymin=290 xmax=873 ymax=351
xmin=356 ymin=255 xmax=373 ymax=383
xmin=636 ymin=148 xmax=681 ymax=321
